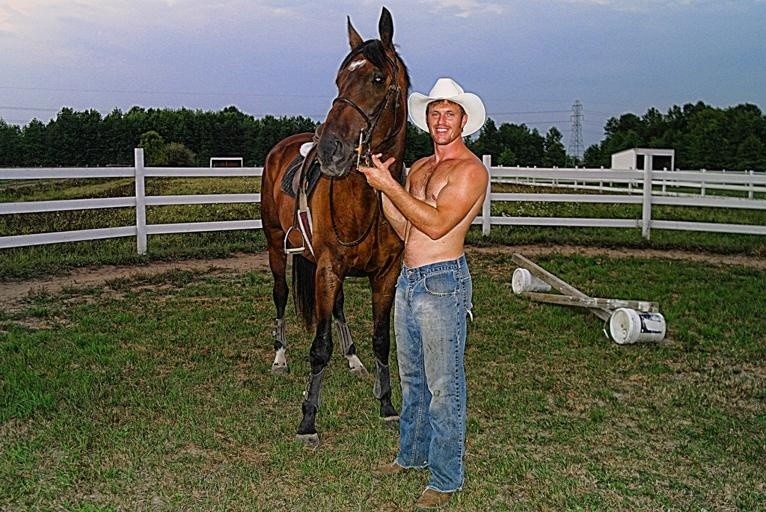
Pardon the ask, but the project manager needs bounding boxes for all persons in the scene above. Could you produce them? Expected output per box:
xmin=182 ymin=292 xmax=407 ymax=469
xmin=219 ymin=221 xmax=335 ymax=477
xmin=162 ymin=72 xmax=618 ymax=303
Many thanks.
xmin=356 ymin=79 xmax=488 ymax=509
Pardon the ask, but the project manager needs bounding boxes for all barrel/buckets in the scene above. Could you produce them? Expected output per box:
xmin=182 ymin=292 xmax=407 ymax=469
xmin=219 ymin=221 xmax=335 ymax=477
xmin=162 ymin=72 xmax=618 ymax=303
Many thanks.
xmin=608 ymin=307 xmax=667 ymax=345
xmin=511 ymin=267 xmax=552 ymax=295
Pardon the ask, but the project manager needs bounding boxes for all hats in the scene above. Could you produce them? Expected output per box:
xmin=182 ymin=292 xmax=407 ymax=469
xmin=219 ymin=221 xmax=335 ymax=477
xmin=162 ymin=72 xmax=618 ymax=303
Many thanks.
xmin=407 ymin=77 xmax=486 ymax=138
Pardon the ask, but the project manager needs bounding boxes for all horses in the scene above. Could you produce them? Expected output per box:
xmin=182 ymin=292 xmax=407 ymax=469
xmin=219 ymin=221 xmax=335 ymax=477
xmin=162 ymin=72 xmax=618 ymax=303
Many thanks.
xmin=259 ymin=6 xmax=413 ymax=441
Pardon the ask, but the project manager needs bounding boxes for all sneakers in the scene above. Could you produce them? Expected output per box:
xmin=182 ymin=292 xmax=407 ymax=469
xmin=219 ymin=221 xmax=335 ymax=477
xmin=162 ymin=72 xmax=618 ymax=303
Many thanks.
xmin=414 ymin=486 xmax=453 ymax=510
xmin=370 ymin=460 xmax=405 ymax=474
